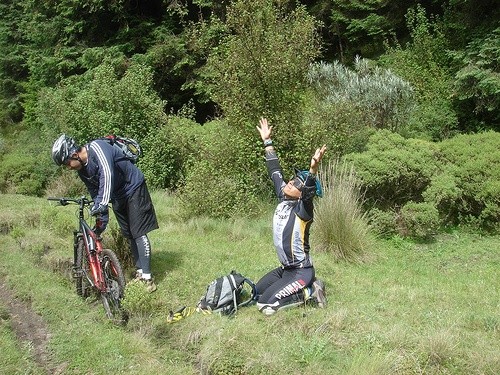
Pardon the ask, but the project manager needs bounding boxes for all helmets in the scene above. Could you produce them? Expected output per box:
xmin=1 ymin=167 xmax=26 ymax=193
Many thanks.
xmin=51 ymin=133 xmax=85 ymax=168
xmin=297 ymin=171 xmax=323 ymax=200
xmin=167 ymin=307 xmax=195 ymax=323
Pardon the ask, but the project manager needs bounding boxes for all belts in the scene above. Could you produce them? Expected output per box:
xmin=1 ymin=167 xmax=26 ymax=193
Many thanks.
xmin=280 ymin=264 xmax=313 ymax=270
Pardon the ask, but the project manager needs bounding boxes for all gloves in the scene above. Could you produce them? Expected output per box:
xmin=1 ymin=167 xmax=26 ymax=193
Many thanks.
xmin=91 ymin=205 xmax=108 ymax=216
xmin=91 ymin=225 xmax=105 ymax=236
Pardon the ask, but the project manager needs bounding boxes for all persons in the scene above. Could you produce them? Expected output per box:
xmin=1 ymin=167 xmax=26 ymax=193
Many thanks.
xmin=251 ymin=116 xmax=327 ymax=316
xmin=52 ymin=133 xmax=159 ymax=295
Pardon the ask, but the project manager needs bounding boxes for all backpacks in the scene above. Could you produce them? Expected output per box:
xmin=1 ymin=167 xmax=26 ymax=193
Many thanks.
xmin=89 ymin=134 xmax=144 ymax=164
xmin=196 ymin=271 xmax=257 ymax=318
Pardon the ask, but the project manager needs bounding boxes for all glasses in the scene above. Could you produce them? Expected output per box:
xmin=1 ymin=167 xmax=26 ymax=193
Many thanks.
xmin=290 ymin=175 xmax=306 ymax=191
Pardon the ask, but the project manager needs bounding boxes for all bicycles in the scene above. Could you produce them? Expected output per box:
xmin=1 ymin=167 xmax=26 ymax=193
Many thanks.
xmin=46 ymin=195 xmax=130 ymax=327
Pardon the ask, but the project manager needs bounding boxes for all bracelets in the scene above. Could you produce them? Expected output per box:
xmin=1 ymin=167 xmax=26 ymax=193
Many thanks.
xmin=265 ymin=140 xmax=273 ymax=146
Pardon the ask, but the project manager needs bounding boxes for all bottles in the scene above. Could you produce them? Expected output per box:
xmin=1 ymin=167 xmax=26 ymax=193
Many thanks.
xmin=88 ymin=234 xmax=97 ymax=253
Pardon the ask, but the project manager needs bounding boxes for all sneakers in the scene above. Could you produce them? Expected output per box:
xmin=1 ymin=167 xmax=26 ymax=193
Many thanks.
xmin=141 ymin=281 xmax=157 ymax=294
xmin=307 ymin=277 xmax=328 ymax=308
xmin=132 ymin=268 xmax=143 ymax=281
